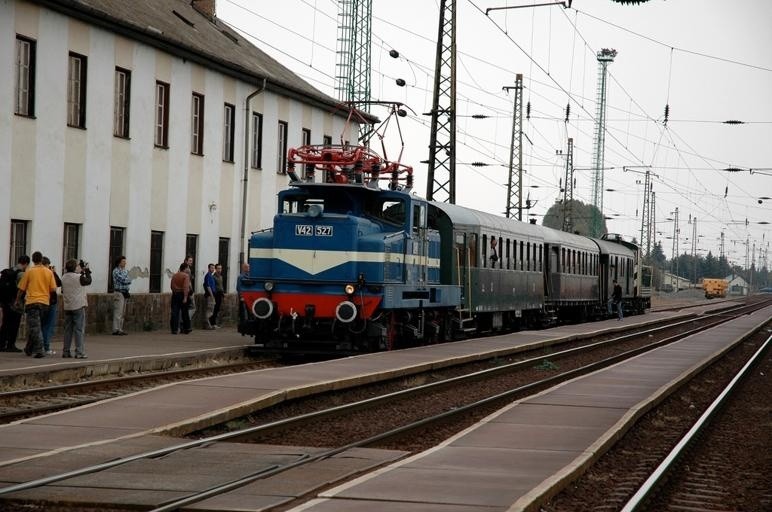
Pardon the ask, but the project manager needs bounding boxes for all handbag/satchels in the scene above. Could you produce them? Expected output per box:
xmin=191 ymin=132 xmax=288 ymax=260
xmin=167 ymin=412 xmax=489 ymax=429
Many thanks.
xmin=123 ymin=290 xmax=130 ymax=299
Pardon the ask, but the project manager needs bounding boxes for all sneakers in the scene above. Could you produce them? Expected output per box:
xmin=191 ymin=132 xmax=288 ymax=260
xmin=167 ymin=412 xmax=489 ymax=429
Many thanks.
xmin=1 ymin=346 xmax=88 ymax=359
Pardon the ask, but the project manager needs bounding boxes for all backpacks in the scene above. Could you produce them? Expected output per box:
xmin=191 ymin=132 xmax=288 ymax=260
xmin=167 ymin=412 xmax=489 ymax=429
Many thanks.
xmin=0 ymin=267 xmax=22 ymax=308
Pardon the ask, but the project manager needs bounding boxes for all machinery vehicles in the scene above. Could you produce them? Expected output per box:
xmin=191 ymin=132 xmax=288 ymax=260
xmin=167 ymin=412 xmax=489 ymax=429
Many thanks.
xmin=702 ymin=278 xmax=729 ymax=299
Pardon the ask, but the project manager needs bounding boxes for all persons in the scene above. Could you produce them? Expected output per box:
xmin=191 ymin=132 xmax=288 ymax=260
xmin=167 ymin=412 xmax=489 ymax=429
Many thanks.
xmin=607 ymin=279 xmax=625 ymax=322
xmin=490 ymin=239 xmax=499 ymax=269
xmin=0 ymin=250 xmax=251 ymax=360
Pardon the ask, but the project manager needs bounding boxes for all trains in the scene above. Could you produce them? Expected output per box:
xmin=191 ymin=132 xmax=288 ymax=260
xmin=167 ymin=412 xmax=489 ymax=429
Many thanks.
xmin=235 ymin=142 xmax=654 ymax=369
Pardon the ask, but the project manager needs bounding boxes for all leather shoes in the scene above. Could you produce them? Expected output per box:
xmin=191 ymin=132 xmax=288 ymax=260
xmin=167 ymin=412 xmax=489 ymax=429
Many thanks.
xmin=171 ymin=324 xmax=223 ymax=334
xmin=112 ymin=331 xmax=123 ymax=336
xmin=121 ymin=331 xmax=128 ymax=335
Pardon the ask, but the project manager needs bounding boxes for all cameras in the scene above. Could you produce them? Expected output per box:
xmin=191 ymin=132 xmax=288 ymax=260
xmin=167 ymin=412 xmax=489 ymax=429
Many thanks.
xmin=49 ymin=265 xmax=55 ymax=269
xmin=80 ymin=258 xmax=88 ymax=269
